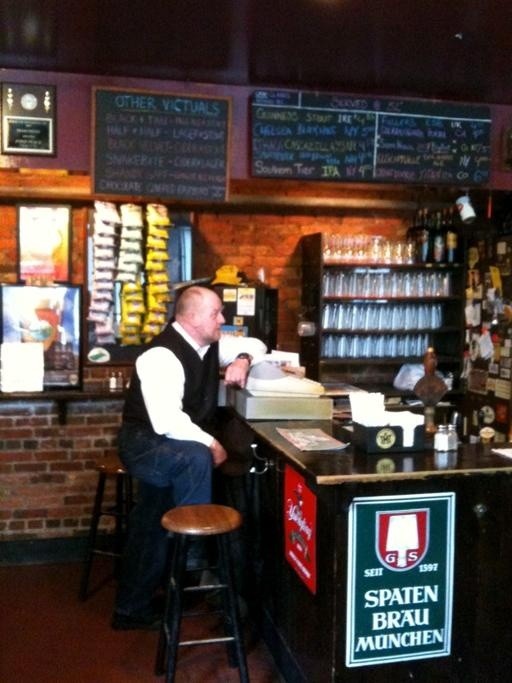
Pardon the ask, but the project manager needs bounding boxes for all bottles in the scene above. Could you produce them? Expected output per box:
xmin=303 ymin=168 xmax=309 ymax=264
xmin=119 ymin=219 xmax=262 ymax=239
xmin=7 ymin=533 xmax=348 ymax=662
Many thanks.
xmin=406 ymin=207 xmax=458 ymax=263
xmin=434 ymin=425 xmax=448 ymax=451
xmin=448 ymin=425 xmax=458 ymax=451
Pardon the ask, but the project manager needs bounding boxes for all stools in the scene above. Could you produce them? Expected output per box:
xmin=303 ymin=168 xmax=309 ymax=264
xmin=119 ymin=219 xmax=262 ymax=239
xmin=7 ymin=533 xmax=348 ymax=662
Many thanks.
xmin=77 ymin=455 xmax=144 ymax=628
xmin=153 ymin=504 xmax=250 ymax=682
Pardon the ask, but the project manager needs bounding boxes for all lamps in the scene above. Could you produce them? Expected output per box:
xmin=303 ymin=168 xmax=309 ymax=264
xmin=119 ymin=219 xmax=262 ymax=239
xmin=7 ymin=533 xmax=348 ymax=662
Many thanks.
xmin=457 ymin=195 xmax=475 ymax=224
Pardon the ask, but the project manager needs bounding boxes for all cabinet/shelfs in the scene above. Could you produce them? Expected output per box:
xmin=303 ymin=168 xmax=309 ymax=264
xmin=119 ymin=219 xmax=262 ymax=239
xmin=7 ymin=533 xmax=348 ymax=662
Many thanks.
xmin=300 ymin=234 xmax=465 ymax=397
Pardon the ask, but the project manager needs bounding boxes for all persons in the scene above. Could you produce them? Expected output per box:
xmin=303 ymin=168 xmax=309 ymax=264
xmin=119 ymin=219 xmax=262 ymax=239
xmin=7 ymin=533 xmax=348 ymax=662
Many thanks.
xmin=108 ymin=284 xmax=268 ymax=631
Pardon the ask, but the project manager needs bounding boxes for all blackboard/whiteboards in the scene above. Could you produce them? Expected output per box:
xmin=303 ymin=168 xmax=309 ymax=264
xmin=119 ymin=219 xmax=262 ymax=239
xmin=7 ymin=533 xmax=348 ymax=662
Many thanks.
xmin=249 ymin=88 xmax=492 ymax=188
xmin=89 ymin=85 xmax=232 ymax=205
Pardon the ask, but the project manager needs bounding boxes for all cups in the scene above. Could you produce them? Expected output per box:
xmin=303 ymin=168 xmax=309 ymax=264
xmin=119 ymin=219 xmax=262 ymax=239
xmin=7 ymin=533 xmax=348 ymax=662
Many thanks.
xmin=321 ymin=301 xmax=446 ymax=331
xmin=321 ymin=331 xmax=432 ymax=363
xmin=324 ymin=267 xmax=454 ymax=298
xmin=323 ymin=232 xmax=416 ymax=264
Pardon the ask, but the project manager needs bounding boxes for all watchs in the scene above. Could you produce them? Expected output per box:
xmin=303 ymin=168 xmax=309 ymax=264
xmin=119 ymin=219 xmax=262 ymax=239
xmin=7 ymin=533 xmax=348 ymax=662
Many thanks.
xmin=236 ymin=353 xmax=252 ymax=365
